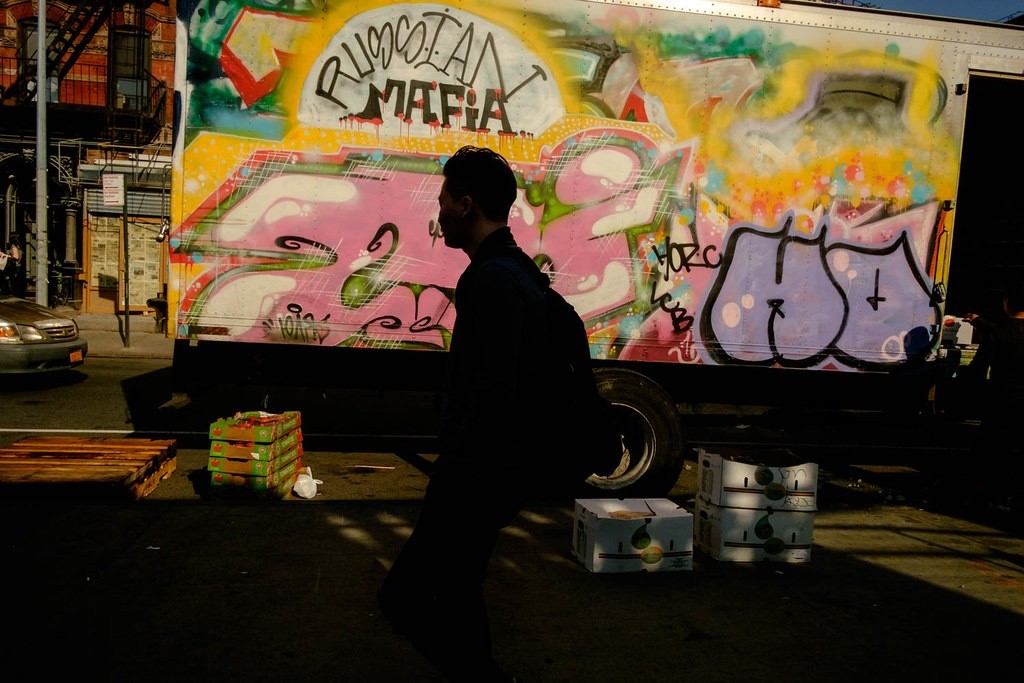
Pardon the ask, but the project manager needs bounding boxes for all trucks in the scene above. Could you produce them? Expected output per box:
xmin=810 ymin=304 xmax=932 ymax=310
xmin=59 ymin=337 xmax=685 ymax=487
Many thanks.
xmin=161 ymin=0 xmax=1024 ymax=498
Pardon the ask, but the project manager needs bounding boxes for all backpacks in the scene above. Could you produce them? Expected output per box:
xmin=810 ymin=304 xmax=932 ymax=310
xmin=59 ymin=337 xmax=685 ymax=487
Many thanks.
xmin=485 ymin=257 xmax=608 ymax=470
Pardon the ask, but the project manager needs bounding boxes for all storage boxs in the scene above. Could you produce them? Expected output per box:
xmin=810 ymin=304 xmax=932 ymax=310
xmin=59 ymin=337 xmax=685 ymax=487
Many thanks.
xmin=697 ymin=446 xmax=818 ymax=512
xmin=207 ymin=443 xmax=304 ymax=476
xmin=573 ymin=498 xmax=693 ymax=572
xmin=210 ymin=426 xmax=304 ymax=461
xmin=209 ymin=409 xmax=302 ymax=443
xmin=942 ymin=314 xmax=974 ymax=345
xmin=694 ymin=498 xmax=814 ymax=563
xmin=209 ymin=457 xmax=302 ymax=488
xmin=212 ymin=474 xmax=299 ymax=503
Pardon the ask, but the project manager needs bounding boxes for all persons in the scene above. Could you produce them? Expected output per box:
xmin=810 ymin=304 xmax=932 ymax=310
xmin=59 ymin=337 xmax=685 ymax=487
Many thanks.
xmin=964 ymin=294 xmax=1024 ymax=512
xmin=376 ymin=144 xmax=542 ymax=683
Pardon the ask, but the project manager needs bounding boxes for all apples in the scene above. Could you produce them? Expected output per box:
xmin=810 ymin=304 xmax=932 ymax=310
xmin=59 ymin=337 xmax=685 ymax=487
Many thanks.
xmin=231 ymin=415 xmax=272 ymax=447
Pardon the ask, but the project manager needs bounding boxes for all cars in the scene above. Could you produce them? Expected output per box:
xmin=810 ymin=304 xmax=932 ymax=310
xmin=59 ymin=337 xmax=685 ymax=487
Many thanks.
xmin=0 ymin=293 xmax=91 ymax=375
